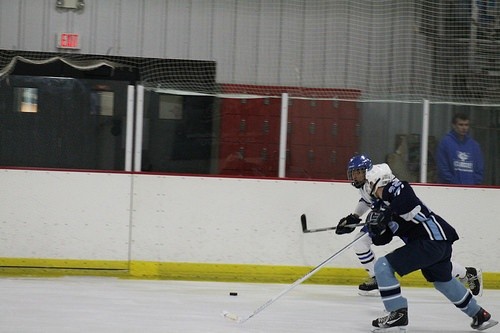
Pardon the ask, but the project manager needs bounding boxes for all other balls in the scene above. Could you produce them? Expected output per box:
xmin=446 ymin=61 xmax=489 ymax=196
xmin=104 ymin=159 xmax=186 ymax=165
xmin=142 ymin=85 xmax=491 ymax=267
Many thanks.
xmin=229 ymin=292 xmax=237 ymax=295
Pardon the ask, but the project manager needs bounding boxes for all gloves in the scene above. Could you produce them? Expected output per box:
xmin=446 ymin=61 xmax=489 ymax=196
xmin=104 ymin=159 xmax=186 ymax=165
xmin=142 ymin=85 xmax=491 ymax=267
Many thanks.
xmin=372 ymin=225 xmax=393 ymax=246
xmin=366 ymin=209 xmax=393 ymax=231
xmin=336 ymin=214 xmax=362 ymax=235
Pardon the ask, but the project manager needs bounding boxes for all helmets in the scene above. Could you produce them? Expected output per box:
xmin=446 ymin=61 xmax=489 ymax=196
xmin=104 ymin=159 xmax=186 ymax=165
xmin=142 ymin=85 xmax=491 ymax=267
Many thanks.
xmin=347 ymin=155 xmax=372 ymax=188
xmin=365 ymin=163 xmax=395 ymax=195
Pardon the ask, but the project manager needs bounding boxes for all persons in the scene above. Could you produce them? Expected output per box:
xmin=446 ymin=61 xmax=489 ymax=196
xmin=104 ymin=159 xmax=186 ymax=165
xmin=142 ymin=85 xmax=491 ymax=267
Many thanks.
xmin=365 ymin=163 xmax=499 ymax=333
xmin=335 ymin=155 xmax=483 ymax=297
xmin=435 ymin=114 xmax=484 ymax=186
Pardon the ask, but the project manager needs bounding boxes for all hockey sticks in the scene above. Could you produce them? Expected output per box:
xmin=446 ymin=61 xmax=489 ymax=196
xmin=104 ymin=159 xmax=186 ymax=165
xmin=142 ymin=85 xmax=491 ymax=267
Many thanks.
xmin=222 ymin=231 xmax=368 ymax=322
xmin=301 ymin=213 xmax=366 ymax=234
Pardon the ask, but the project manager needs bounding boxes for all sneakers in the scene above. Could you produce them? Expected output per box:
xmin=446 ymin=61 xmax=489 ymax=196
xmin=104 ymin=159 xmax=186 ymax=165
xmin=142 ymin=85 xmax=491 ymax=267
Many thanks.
xmin=466 ymin=267 xmax=483 ymax=296
xmin=372 ymin=311 xmax=408 ymax=332
xmin=470 ymin=308 xmax=499 ymax=331
xmin=359 ymin=282 xmax=381 ymax=296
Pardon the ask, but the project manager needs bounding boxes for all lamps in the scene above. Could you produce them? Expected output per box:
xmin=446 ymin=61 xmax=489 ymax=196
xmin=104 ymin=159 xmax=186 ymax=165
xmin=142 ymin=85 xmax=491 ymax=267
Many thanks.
xmin=55 ymin=0 xmax=84 ymax=13
